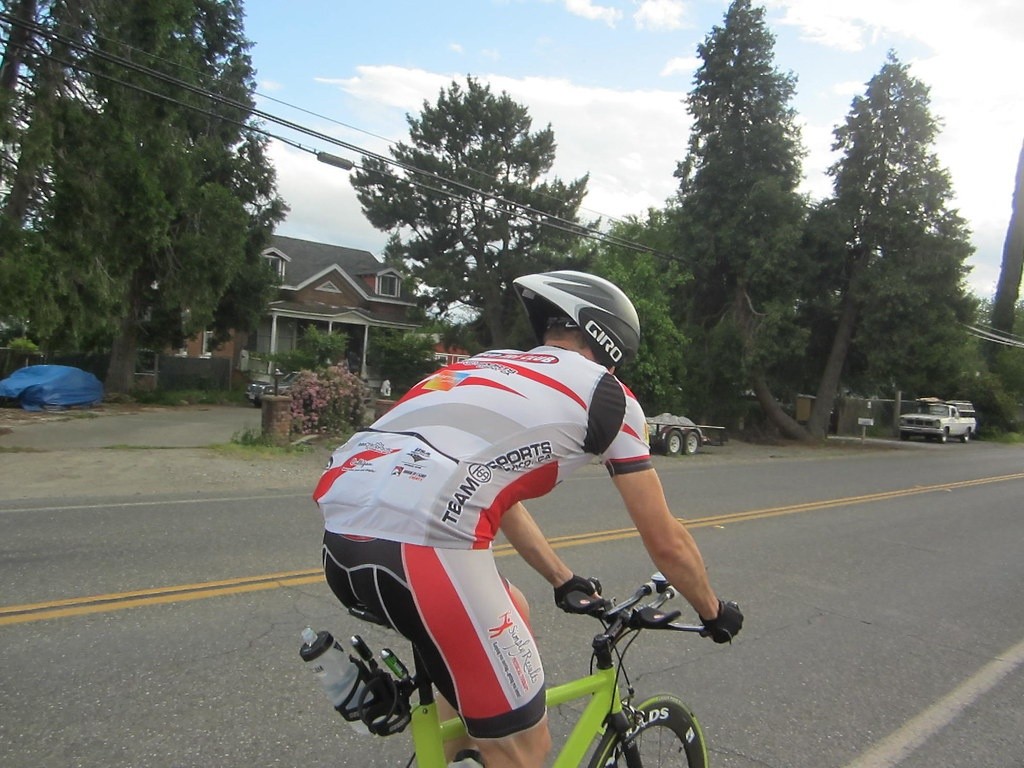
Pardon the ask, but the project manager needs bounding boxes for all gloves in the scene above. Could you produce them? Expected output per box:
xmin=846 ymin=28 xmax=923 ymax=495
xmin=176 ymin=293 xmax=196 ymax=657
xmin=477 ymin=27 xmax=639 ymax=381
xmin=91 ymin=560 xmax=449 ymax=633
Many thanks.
xmin=554 ymin=574 xmax=595 ymax=613
xmin=699 ymin=598 xmax=743 ymax=644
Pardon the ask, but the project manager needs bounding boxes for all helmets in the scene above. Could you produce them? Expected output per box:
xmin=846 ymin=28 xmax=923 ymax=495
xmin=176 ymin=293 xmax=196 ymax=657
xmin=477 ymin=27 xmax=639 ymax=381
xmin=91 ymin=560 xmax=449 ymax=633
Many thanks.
xmin=513 ymin=269 xmax=641 ymax=374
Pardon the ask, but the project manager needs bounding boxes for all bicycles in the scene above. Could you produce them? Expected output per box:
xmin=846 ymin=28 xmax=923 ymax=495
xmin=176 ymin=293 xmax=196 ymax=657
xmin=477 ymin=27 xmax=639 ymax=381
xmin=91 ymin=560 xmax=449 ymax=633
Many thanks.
xmin=346 ymin=569 xmax=730 ymax=768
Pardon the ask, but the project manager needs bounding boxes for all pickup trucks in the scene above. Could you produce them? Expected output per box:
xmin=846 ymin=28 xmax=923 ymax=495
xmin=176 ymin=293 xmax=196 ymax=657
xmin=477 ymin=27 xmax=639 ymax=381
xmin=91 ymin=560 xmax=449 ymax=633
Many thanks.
xmin=898 ymin=399 xmax=977 ymax=443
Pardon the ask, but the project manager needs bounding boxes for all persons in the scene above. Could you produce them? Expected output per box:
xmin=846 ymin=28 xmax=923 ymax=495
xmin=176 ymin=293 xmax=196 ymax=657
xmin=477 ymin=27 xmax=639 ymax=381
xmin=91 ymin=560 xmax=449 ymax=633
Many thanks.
xmin=310 ymin=268 xmax=745 ymax=768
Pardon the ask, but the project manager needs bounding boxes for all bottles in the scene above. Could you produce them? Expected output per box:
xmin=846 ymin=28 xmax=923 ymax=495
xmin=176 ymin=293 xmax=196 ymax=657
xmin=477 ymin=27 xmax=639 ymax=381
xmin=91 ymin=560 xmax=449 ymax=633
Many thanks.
xmin=297 ymin=625 xmax=386 ymax=736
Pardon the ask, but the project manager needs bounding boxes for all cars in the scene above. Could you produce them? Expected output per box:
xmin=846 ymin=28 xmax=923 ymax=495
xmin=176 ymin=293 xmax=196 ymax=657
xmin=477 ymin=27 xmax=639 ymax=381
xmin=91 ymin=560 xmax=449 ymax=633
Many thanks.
xmin=0 ymin=364 xmax=104 ymax=409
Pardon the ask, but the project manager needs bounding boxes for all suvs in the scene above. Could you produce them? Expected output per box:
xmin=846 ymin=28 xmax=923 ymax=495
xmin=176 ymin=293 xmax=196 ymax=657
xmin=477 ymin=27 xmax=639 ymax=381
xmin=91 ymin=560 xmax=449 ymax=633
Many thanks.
xmin=246 ymin=369 xmax=312 ymax=407
